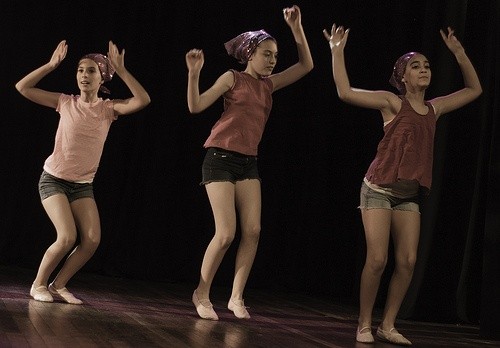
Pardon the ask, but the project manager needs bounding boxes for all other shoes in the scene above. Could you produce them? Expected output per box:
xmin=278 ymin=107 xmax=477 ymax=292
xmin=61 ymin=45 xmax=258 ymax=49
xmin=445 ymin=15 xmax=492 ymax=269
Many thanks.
xmin=192 ymin=290 xmax=218 ymax=320
xmin=30 ymin=284 xmax=83 ymax=304
xmin=356 ymin=326 xmax=374 ymax=342
xmin=228 ymin=298 xmax=250 ymax=318
xmin=376 ymin=327 xmax=411 ymax=345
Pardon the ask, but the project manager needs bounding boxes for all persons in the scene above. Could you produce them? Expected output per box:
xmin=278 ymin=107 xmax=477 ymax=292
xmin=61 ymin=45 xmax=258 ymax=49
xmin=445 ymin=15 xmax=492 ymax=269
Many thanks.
xmin=186 ymin=4 xmax=314 ymax=320
xmin=16 ymin=40 xmax=151 ymax=305
xmin=323 ymin=22 xmax=482 ymax=345
